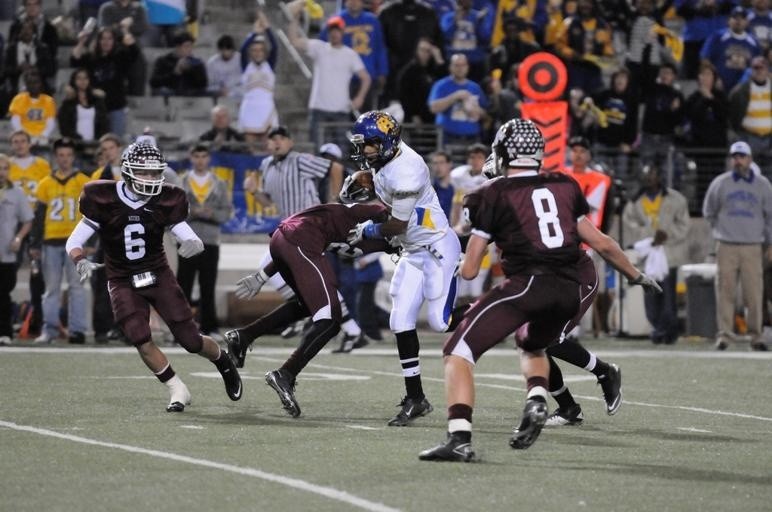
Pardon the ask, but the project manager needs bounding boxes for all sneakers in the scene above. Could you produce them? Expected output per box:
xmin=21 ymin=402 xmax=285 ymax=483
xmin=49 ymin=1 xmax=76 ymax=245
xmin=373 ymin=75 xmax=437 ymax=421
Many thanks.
xmin=509 ymin=395 xmax=548 ymax=450
xmin=545 ymin=404 xmax=584 ymax=427
xmin=650 ymin=327 xmax=769 ymax=350
xmin=165 ymin=385 xmax=191 ymax=412
xmin=332 ymin=329 xmax=367 ymax=354
xmin=264 ymin=370 xmax=301 ymax=418
xmin=418 ymin=440 xmax=475 ymax=461
xmin=223 ymin=328 xmax=248 ymax=368
xmin=387 ymin=397 xmax=434 ymax=426
xmin=598 ymin=363 xmax=622 ymax=416
xmin=281 ymin=323 xmax=301 ymax=338
xmin=0 ymin=331 xmax=225 ymax=345
xmin=218 ymin=350 xmax=242 ymax=401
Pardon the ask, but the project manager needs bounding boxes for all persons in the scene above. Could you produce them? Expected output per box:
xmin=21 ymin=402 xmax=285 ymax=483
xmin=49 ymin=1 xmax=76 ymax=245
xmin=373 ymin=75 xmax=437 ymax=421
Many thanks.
xmin=453 ymin=150 xmax=623 ymax=429
xmin=222 ymin=202 xmax=403 ymax=418
xmin=0 ymin=1 xmax=772 ymax=353
xmin=418 ymin=117 xmax=664 ymax=464
xmin=65 ymin=142 xmax=243 ymax=414
xmin=338 ymin=110 xmax=475 ymax=429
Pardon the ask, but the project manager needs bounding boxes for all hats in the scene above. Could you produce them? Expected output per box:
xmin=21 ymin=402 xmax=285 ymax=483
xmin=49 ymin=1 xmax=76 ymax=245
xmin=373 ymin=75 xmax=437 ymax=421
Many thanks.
xmin=728 ymin=140 xmax=752 ymax=156
xmin=267 ymin=125 xmax=290 ymax=138
xmin=567 ymin=136 xmax=591 ymax=148
xmin=730 ymin=7 xmax=748 ymax=16
xmin=319 ymin=143 xmax=342 ymax=159
xmin=326 ymin=16 xmax=346 ymax=29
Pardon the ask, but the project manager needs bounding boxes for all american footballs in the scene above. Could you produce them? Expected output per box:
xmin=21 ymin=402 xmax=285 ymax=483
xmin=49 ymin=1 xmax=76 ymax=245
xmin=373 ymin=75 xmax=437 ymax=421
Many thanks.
xmin=351 ymin=169 xmax=374 ymax=200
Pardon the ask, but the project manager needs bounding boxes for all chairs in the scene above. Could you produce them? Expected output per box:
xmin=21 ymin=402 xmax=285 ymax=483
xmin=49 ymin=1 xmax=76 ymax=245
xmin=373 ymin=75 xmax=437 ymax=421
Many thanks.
xmin=682 ymin=78 xmax=698 ymax=102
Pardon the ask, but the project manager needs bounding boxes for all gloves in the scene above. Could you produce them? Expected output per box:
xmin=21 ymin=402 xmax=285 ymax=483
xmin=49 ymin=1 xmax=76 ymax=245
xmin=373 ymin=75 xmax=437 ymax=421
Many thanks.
xmin=650 ymin=229 xmax=667 ymax=247
xmin=178 ymin=239 xmax=204 ymax=259
xmin=234 ymin=268 xmax=269 ymax=301
xmin=338 ymin=175 xmax=370 ymax=204
xmin=345 ymin=219 xmax=373 ymax=246
xmin=629 ymin=273 xmax=664 ymax=295
xmin=75 ymin=258 xmax=106 ymax=283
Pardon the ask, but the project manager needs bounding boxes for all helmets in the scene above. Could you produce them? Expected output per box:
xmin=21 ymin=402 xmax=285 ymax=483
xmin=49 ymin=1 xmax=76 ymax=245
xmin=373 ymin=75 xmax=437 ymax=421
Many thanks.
xmin=492 ymin=119 xmax=545 ymax=176
xmin=121 ymin=142 xmax=165 ymax=171
xmin=348 ymin=110 xmax=398 ymax=144
xmin=481 ymin=157 xmax=496 ymax=178
